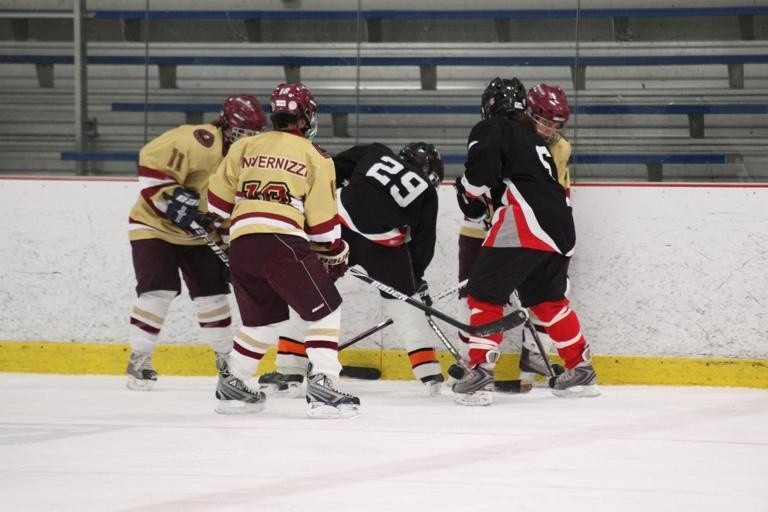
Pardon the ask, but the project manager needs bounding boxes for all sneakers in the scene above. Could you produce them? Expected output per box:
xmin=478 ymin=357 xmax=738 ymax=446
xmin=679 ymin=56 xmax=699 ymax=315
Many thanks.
xmin=258 ymin=371 xmax=305 ymax=384
xmin=421 ymin=374 xmax=446 ymax=381
xmin=126 ymin=350 xmax=159 ymax=381
xmin=306 ymin=374 xmax=361 ymax=407
xmin=549 ymin=364 xmax=599 ymax=389
xmin=452 ymin=367 xmax=495 ymax=392
xmin=216 ymin=371 xmax=267 ymax=405
xmin=217 ymin=357 xmax=229 ymax=372
xmin=521 ymin=348 xmax=564 ymax=376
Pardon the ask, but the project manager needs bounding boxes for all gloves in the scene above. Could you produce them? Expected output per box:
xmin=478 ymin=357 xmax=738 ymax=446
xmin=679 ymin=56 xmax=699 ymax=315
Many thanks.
xmin=213 ymin=243 xmax=228 ymax=282
xmin=317 ymin=241 xmax=349 ymax=280
xmin=457 ymin=190 xmax=488 ymax=223
xmin=200 ymin=212 xmax=229 ymax=236
xmin=166 ymin=188 xmax=199 ymax=229
xmin=417 ymin=279 xmax=432 ymax=307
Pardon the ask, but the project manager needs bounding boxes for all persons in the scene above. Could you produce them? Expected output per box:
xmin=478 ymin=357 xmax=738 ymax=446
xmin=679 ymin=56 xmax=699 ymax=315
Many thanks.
xmin=207 ymin=83 xmax=362 ymax=419
xmin=332 ymin=140 xmax=445 ymax=396
xmin=450 ymin=76 xmax=601 ymax=406
xmin=127 ymin=94 xmax=267 ymax=389
xmin=522 ymin=83 xmax=572 ymax=378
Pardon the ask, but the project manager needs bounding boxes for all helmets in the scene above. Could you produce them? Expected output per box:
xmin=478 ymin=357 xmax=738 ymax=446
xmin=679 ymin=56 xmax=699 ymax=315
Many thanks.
xmin=527 ymin=82 xmax=570 ymax=145
xmin=399 ymin=143 xmax=445 ymax=188
xmin=481 ymin=76 xmax=528 ymax=119
xmin=219 ymin=94 xmax=266 ymax=148
xmin=270 ymin=82 xmax=319 ymax=141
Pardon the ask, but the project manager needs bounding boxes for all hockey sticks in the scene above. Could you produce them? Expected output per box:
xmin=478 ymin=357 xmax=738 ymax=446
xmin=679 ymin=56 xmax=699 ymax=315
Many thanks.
xmin=344 ymin=262 xmax=528 ymax=338
xmin=163 ymin=192 xmax=381 ymax=379
xmin=483 ymin=215 xmax=561 ymax=389
xmin=426 ymin=315 xmax=533 ymax=394
